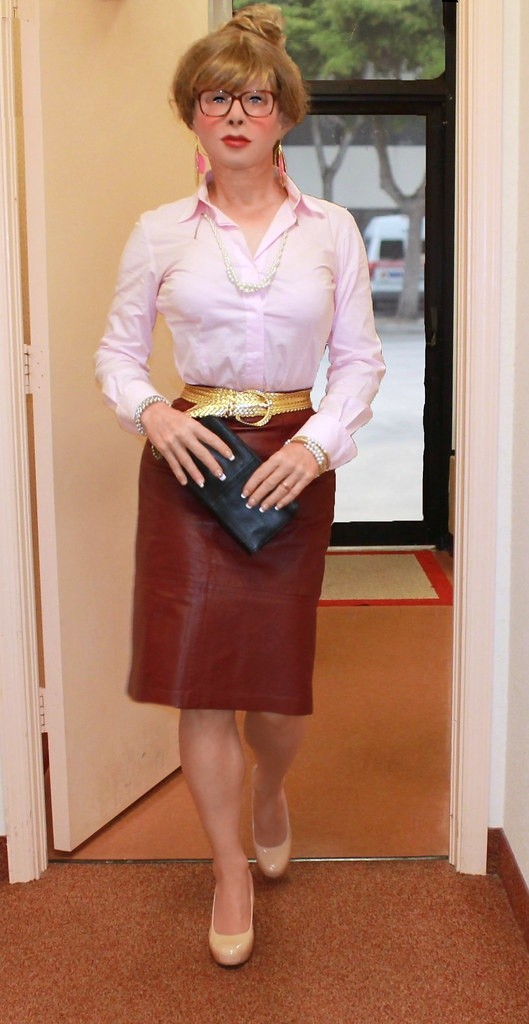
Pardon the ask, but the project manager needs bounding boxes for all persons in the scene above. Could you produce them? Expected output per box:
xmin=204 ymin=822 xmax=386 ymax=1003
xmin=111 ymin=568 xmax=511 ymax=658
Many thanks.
xmin=93 ymin=6 xmax=386 ymax=965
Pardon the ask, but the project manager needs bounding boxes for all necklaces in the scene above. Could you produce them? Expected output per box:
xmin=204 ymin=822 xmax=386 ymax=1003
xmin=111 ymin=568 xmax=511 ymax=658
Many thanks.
xmin=203 ymin=213 xmax=288 ymax=293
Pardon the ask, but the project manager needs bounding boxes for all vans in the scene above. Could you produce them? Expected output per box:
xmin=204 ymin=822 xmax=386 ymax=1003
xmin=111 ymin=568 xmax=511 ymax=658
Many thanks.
xmin=362 ymin=214 xmax=425 ymax=307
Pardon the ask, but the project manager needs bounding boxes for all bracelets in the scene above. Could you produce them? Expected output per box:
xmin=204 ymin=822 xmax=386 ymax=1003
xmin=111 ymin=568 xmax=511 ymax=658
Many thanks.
xmin=135 ymin=397 xmax=171 ymax=437
xmin=285 ymin=435 xmax=332 ymax=479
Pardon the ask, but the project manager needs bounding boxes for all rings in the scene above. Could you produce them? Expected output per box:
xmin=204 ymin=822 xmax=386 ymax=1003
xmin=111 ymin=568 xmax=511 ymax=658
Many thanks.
xmin=282 ymin=481 xmax=290 ymax=491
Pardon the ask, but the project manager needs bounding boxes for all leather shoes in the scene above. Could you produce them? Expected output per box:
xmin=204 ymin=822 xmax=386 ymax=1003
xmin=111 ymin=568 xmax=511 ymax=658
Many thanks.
xmin=251 ymin=786 xmax=291 ymax=878
xmin=208 ymin=869 xmax=255 ymax=970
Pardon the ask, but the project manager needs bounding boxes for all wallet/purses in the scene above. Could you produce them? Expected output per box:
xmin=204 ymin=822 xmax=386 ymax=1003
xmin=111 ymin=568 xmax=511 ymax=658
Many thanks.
xmin=175 ymin=414 xmax=301 ymax=554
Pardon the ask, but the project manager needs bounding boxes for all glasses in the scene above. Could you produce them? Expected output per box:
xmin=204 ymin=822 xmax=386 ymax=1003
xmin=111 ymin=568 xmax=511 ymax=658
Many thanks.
xmin=193 ymin=90 xmax=282 ymax=118
